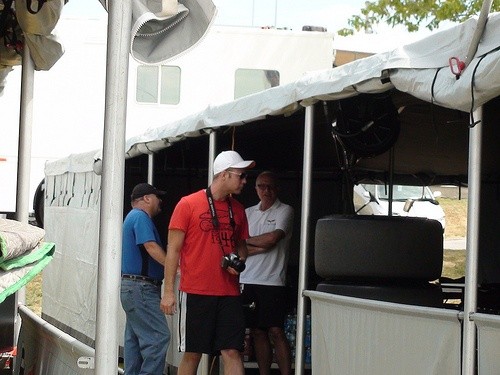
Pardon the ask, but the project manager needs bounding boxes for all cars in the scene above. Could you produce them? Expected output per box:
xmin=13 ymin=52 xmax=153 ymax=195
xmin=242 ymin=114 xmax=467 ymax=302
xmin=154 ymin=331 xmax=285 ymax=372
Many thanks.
xmin=352 ymin=183 xmax=446 ymax=234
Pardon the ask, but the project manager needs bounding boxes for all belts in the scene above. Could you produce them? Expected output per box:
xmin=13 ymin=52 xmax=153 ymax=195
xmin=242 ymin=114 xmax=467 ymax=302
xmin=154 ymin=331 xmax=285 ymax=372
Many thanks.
xmin=122 ymin=275 xmax=163 ymax=285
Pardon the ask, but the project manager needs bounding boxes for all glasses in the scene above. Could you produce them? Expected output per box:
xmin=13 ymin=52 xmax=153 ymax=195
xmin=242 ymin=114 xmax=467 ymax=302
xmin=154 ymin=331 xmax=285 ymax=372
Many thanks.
xmin=256 ymin=183 xmax=277 ymax=190
xmin=226 ymin=172 xmax=246 ymax=179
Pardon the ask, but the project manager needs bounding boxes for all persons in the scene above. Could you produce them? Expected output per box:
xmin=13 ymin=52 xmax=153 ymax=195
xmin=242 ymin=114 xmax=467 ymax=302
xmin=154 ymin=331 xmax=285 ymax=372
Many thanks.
xmin=239 ymin=171 xmax=295 ymax=375
xmin=160 ymin=151 xmax=249 ymax=375
xmin=120 ymin=183 xmax=180 ymax=375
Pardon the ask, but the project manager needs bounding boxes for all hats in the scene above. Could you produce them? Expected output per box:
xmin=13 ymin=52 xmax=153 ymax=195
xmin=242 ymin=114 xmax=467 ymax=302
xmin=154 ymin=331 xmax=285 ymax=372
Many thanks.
xmin=210 ymin=150 xmax=256 ymax=179
xmin=130 ymin=183 xmax=168 ymax=201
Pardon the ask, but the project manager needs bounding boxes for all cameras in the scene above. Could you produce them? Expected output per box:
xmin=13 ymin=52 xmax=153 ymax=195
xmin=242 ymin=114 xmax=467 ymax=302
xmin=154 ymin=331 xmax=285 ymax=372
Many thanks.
xmin=220 ymin=252 xmax=246 ymax=272
